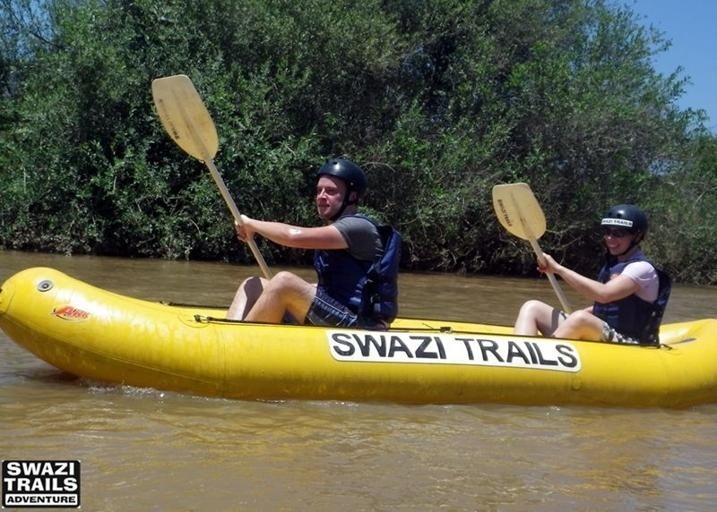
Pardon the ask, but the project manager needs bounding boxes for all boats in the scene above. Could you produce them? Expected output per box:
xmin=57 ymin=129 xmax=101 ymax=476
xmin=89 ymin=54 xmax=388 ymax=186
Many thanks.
xmin=0 ymin=265 xmax=716 ymax=411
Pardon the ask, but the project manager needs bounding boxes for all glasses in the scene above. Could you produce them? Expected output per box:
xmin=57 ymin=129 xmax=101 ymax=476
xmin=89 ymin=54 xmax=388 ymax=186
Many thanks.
xmin=601 ymin=228 xmax=628 ymax=238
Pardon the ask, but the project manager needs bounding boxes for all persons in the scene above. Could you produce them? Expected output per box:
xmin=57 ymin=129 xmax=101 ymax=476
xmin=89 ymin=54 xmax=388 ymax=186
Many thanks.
xmin=223 ymin=159 xmax=402 ymax=332
xmin=513 ymin=204 xmax=672 ymax=348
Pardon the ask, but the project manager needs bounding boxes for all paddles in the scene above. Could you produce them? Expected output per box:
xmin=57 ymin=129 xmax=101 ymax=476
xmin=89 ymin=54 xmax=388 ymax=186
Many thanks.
xmin=491 ymin=183 xmax=574 ymax=314
xmin=151 ymin=74 xmax=272 ymax=280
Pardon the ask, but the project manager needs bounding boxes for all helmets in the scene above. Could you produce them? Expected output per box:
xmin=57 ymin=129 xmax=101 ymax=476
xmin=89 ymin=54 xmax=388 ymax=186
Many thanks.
xmin=316 ymin=158 xmax=368 ymax=198
xmin=598 ymin=204 xmax=648 ymax=232
